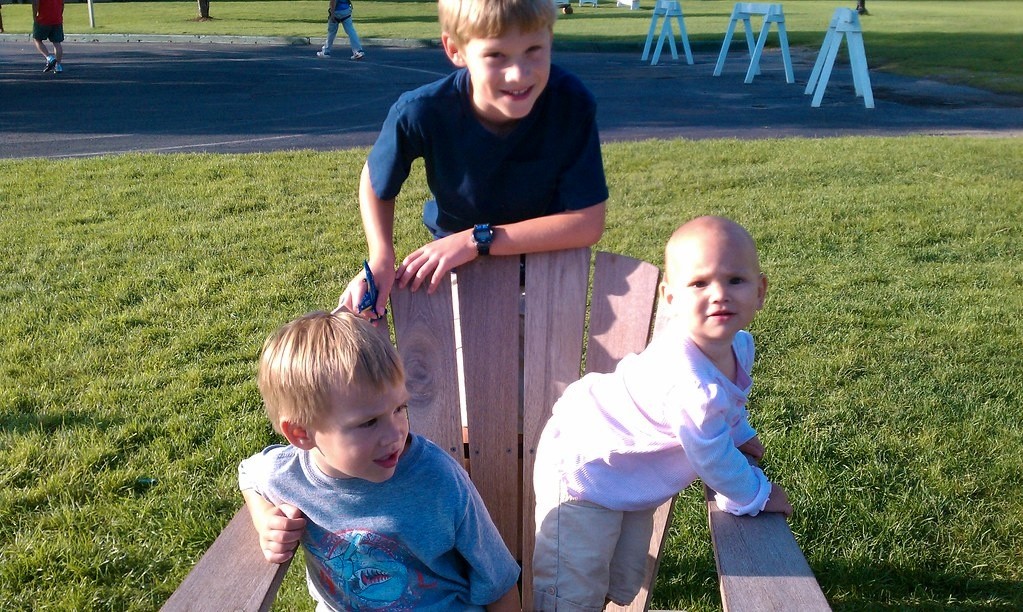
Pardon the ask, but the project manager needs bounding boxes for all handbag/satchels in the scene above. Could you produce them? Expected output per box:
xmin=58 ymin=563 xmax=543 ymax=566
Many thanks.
xmin=332 ymin=6 xmax=352 ymax=22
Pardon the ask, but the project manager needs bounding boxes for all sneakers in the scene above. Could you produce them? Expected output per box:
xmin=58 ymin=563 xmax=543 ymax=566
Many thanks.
xmin=317 ymin=51 xmax=331 ymax=59
xmin=43 ymin=54 xmax=56 ymax=72
xmin=54 ymin=63 xmax=62 ymax=73
xmin=351 ymin=49 xmax=365 ymax=59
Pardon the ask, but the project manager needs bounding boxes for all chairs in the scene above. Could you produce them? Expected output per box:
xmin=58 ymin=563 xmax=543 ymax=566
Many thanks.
xmin=157 ymin=249 xmax=838 ymax=612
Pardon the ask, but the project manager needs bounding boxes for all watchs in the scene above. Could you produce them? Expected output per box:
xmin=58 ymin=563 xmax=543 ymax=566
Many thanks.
xmin=471 ymin=223 xmax=495 ymax=257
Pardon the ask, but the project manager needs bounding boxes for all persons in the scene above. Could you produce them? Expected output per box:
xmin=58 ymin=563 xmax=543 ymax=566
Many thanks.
xmin=338 ymin=0 xmax=610 ymax=328
xmin=238 ymin=312 xmax=521 ymax=612
xmin=316 ymin=0 xmax=365 ymax=60
xmin=531 ymin=215 xmax=794 ymax=612
xmin=31 ymin=0 xmax=65 ymax=75
